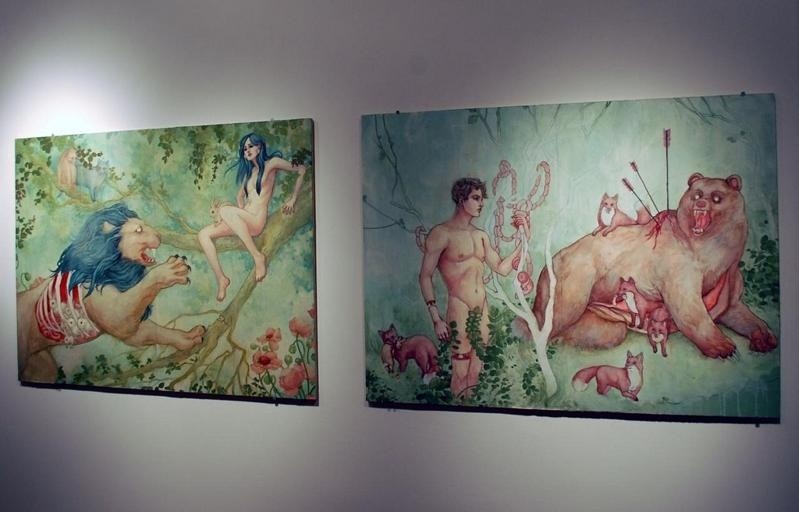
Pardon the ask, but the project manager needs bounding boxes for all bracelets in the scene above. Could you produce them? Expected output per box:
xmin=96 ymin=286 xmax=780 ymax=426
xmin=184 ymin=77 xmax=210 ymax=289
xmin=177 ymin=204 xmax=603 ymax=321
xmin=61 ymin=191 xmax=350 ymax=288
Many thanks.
xmin=425 ymin=300 xmax=437 ymax=308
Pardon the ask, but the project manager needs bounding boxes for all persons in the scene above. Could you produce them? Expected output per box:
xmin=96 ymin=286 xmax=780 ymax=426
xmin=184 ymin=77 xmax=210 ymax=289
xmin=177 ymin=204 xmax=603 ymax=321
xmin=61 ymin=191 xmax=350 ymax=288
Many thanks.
xmin=200 ymin=134 xmax=306 ymax=302
xmin=419 ymin=176 xmax=531 ymax=397
